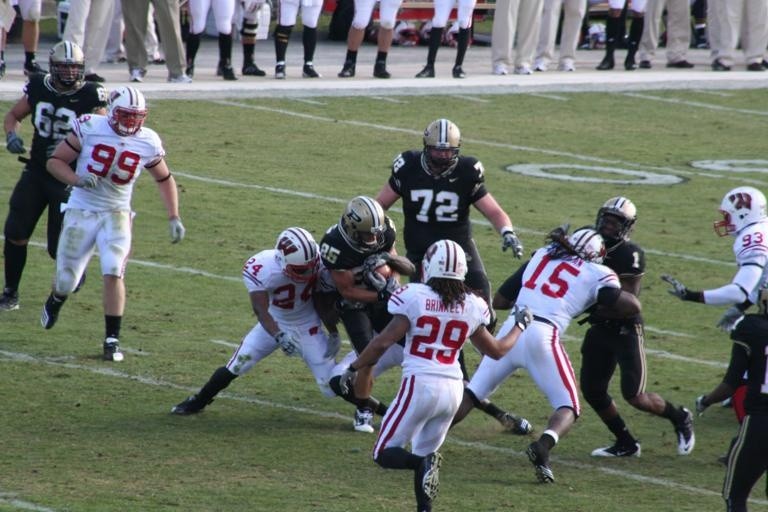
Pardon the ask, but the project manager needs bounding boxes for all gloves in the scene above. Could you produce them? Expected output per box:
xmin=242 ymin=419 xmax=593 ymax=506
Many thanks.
xmin=660 ymin=274 xmax=704 ymax=303
xmin=697 ymin=396 xmax=707 ymax=418
xmin=340 ymin=364 xmax=359 ymax=395
xmin=717 ymin=299 xmax=754 ymax=333
xmin=75 ymin=172 xmax=98 ymax=188
xmin=323 ymin=333 xmax=341 ymax=359
xmin=169 ymin=216 xmax=184 ymax=243
xmin=6 ymin=131 xmax=26 ymax=153
xmin=514 ymin=304 xmax=532 ymax=332
xmin=273 ymin=330 xmax=300 ymax=357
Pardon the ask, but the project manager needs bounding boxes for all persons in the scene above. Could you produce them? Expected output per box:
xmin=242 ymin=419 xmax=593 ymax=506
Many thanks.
xmin=696 ymin=280 xmax=768 ymax=512
xmin=1 ymin=39 xmax=110 ymax=310
xmin=376 ymin=117 xmax=522 ymax=315
xmin=339 ymin=239 xmax=533 ymax=512
xmin=2 ymin=1 xmax=766 ymax=81
xmin=561 ymin=195 xmax=695 ymax=458
xmin=452 ymin=228 xmax=642 ymax=483
xmin=661 ymin=183 xmax=768 ymax=380
xmin=320 ymin=196 xmax=410 ymax=434
xmin=40 ymin=87 xmax=186 ymax=362
xmin=171 ymin=225 xmax=342 ymax=416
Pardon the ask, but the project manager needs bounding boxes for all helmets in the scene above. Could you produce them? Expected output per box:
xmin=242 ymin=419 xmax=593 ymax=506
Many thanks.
xmin=106 ymin=86 xmax=148 ymax=135
xmin=721 ymin=185 xmax=768 ymax=236
xmin=588 ymin=23 xmax=608 ymax=49
xmin=273 ymin=195 xmax=465 ymax=285
xmin=423 ymin=119 xmax=461 ymax=149
xmin=572 ymin=196 xmax=637 ymax=261
xmin=49 ymin=40 xmax=85 ymax=91
xmin=370 ymin=21 xmax=472 ymax=48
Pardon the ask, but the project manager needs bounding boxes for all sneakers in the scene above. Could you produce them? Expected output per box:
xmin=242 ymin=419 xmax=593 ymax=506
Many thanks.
xmin=421 ymin=450 xmax=444 ymax=502
xmin=526 ymin=440 xmax=555 ymax=486
xmin=511 ymin=416 xmax=535 ymax=436
xmin=352 ymin=409 xmax=375 ymax=434
xmin=1 ymin=272 xmax=124 ymax=361
xmin=172 ymin=393 xmax=203 ymax=423
xmin=672 ymin=408 xmax=695 ymax=455
xmin=591 ymin=437 xmax=640 ymax=458
xmin=1 ymin=57 xmax=265 ymax=82
xmin=493 ymin=54 xmax=766 ymax=75
xmin=274 ymin=61 xmax=466 ymax=78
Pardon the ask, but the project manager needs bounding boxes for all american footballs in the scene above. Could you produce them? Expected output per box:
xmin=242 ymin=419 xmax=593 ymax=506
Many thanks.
xmin=375 ymin=264 xmax=391 ymax=280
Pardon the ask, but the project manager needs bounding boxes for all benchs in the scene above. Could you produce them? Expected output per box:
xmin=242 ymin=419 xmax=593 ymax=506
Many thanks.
xmin=320 ymin=0 xmax=648 ymax=44
